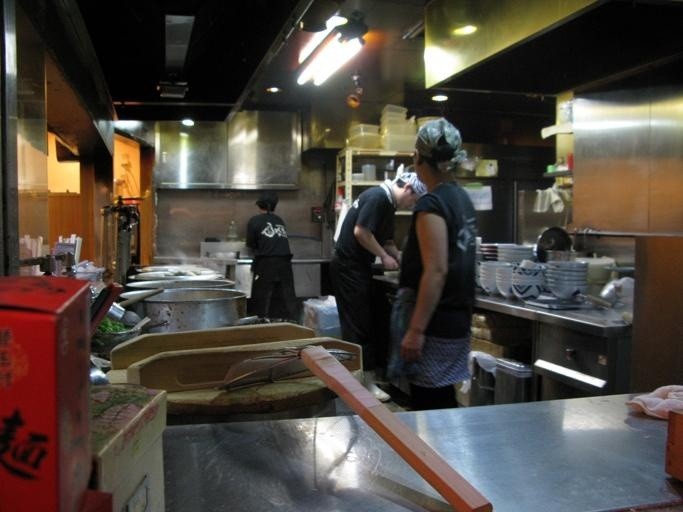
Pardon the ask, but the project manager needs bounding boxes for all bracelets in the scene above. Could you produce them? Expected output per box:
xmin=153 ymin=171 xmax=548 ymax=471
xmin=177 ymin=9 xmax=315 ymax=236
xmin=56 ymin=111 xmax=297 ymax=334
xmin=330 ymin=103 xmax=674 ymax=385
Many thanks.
xmin=407 ymin=324 xmax=424 ymax=336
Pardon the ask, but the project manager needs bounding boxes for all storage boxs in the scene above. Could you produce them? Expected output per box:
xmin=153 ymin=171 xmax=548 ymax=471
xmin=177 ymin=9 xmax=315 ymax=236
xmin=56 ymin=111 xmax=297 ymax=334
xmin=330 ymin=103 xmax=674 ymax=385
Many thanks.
xmin=0 ymin=275 xmax=165 ymax=512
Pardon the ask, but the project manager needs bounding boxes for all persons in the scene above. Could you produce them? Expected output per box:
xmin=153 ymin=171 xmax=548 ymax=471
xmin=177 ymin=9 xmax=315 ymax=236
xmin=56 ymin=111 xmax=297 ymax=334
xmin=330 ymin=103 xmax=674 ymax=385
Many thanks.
xmin=383 ymin=118 xmax=478 ymax=408
xmin=327 ymin=171 xmax=428 ymax=404
xmin=245 ymin=190 xmax=301 ymax=324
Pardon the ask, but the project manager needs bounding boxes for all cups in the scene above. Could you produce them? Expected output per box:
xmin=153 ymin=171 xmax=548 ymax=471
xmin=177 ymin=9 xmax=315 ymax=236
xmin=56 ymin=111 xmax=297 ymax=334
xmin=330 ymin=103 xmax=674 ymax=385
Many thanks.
xmin=362 ymin=164 xmax=376 ymax=181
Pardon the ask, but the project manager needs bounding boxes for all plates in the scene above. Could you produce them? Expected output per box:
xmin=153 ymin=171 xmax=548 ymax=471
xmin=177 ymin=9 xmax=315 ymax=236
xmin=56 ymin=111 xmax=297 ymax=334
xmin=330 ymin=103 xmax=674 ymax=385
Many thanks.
xmin=479 ymin=244 xmax=534 ymax=261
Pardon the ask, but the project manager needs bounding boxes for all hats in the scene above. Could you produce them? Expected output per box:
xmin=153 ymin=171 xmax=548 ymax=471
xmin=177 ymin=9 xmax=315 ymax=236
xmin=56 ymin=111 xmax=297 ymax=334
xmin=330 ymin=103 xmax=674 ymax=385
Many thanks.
xmin=256 ymin=194 xmax=280 ymax=211
xmin=398 ymin=173 xmax=427 ymax=194
xmin=414 ymin=117 xmax=467 ymax=170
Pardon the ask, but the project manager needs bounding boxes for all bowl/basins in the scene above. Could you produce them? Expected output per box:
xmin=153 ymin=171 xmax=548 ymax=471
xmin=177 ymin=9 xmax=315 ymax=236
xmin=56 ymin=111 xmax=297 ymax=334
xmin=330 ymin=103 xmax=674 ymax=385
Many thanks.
xmin=479 ymin=260 xmax=589 ymax=302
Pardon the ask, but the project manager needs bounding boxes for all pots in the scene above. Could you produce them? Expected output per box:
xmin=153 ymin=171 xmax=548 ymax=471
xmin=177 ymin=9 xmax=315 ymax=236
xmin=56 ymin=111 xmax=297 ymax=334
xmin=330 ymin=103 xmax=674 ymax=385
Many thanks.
xmin=119 ymin=264 xmax=258 ymax=329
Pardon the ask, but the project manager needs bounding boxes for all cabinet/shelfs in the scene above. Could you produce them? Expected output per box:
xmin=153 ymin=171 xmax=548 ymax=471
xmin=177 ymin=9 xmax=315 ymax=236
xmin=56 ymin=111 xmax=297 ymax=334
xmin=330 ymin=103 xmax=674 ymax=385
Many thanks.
xmin=335 ymin=148 xmax=415 ymax=252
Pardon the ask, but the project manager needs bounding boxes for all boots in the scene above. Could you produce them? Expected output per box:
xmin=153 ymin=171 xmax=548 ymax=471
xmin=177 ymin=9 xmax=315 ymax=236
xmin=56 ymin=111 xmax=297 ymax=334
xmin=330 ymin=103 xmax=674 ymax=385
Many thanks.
xmin=364 ymin=371 xmax=391 ymax=402
